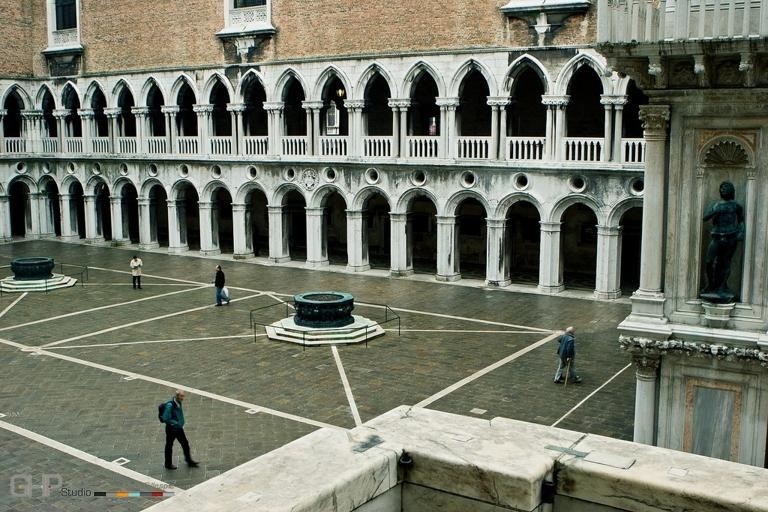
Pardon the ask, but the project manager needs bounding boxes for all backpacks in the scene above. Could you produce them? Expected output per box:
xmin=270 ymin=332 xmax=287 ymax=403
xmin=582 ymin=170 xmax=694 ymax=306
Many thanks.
xmin=158 ymin=400 xmax=177 ymax=423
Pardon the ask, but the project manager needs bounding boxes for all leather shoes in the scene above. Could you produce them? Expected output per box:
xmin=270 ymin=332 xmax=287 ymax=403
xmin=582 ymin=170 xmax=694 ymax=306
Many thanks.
xmin=165 ymin=462 xmax=199 ymax=470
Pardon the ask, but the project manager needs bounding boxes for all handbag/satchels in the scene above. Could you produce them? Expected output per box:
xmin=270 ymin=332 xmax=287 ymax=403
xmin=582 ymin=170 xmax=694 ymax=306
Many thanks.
xmin=556 ymin=346 xmax=562 ymax=354
xmin=223 ymin=287 xmax=228 ymax=297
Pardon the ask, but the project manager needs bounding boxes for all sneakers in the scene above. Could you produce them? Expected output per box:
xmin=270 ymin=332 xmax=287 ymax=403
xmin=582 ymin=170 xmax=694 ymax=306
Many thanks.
xmin=554 ymin=375 xmax=582 ymax=384
xmin=215 ymin=298 xmax=231 ymax=306
xmin=133 ymin=287 xmax=143 ymax=290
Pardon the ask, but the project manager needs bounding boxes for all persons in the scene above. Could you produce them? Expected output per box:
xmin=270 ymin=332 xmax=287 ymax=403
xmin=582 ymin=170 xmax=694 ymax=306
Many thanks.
xmin=554 ymin=327 xmax=584 ymax=384
xmin=211 ymin=264 xmax=231 ymax=306
xmin=703 ymin=182 xmax=746 ymax=294
xmin=130 ymin=255 xmax=143 ymax=290
xmin=160 ymin=390 xmax=201 ymax=470
